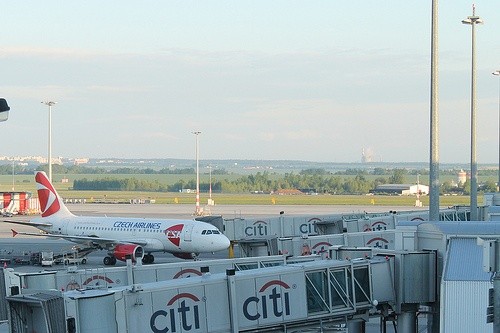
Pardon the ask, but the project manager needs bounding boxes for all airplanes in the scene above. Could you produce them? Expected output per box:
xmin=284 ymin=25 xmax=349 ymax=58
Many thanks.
xmin=0 ymin=168 xmax=232 ymax=267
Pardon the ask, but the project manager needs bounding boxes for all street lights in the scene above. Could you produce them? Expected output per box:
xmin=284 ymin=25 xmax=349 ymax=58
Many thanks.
xmin=41 ymin=101 xmax=57 ymax=186
xmin=461 ymin=4 xmax=484 ymax=224
xmin=191 ymin=131 xmax=202 ymax=215
